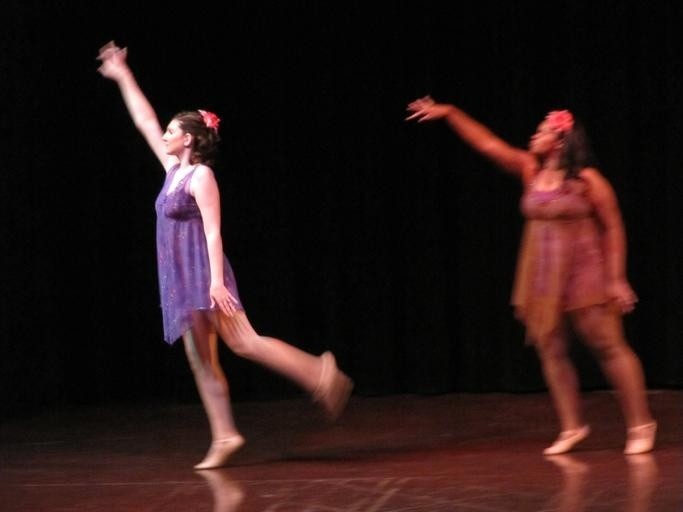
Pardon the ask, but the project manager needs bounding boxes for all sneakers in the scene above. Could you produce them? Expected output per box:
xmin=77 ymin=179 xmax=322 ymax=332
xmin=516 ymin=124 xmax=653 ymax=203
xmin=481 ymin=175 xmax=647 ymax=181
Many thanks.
xmin=542 ymin=425 xmax=591 ymax=456
xmin=623 ymin=421 xmax=658 ymax=455
xmin=193 ymin=434 xmax=245 ymax=470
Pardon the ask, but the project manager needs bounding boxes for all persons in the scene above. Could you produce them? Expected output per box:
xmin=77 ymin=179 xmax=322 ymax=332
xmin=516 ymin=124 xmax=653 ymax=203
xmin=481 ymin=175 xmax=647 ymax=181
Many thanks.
xmin=405 ymin=95 xmax=658 ymax=455
xmin=97 ymin=39 xmax=356 ymax=471
